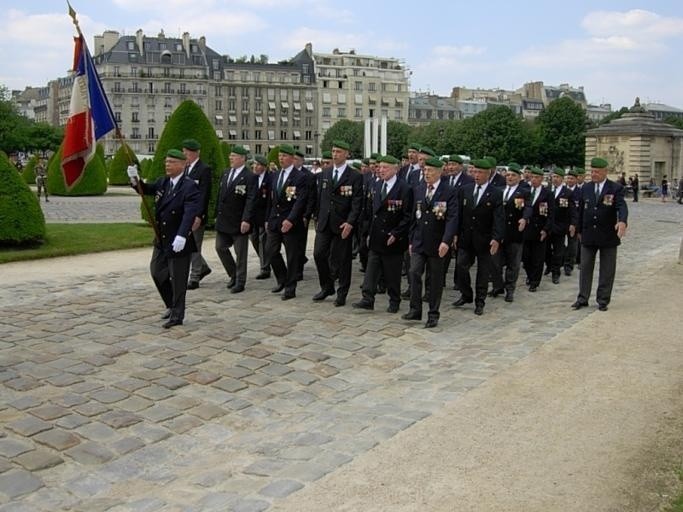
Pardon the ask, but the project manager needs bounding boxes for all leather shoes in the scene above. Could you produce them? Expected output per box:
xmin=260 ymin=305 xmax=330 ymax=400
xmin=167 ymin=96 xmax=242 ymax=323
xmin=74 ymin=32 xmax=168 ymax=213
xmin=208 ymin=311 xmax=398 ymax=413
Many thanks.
xmin=199 ymin=269 xmax=210 ymax=279
xmin=572 ymin=301 xmax=588 ymax=306
xmin=187 ymin=282 xmax=199 ymax=289
xmin=256 ymin=272 xmax=303 ymax=300
xmin=599 ymin=304 xmax=607 ymax=311
xmin=452 ymin=267 xmax=571 ymax=315
xmin=163 ymin=321 xmax=182 ymax=329
xmin=227 ymin=278 xmax=244 ymax=292
xmin=313 ymin=285 xmax=437 ymax=328
xmin=162 ymin=310 xmax=171 ymax=319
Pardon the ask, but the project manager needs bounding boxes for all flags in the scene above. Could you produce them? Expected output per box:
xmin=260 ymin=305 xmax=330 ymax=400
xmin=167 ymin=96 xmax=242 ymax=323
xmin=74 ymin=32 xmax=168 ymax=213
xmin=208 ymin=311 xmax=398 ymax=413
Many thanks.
xmin=58 ymin=34 xmax=120 ymax=196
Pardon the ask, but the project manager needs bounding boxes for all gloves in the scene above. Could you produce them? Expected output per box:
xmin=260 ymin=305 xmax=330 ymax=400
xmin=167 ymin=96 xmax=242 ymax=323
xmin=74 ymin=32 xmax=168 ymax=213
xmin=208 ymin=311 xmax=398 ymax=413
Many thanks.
xmin=172 ymin=236 xmax=186 ymax=252
xmin=127 ymin=165 xmax=138 ymax=178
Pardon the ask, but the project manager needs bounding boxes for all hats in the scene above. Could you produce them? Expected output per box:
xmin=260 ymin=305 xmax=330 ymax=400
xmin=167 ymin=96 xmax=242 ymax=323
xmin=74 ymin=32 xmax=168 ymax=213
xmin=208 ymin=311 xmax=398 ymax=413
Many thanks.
xmin=231 ymin=143 xmax=304 ymax=165
xmin=168 ymin=149 xmax=186 ymax=160
xmin=353 ymin=153 xmax=399 ymax=170
xmin=333 ymin=140 xmax=350 ymax=150
xmin=592 ymin=158 xmax=607 ymax=168
xmin=507 ymin=162 xmax=585 ymax=176
xmin=322 ymin=151 xmax=333 ymax=159
xmin=182 ymin=139 xmax=201 ymax=151
xmin=402 ymin=143 xmax=496 ymax=169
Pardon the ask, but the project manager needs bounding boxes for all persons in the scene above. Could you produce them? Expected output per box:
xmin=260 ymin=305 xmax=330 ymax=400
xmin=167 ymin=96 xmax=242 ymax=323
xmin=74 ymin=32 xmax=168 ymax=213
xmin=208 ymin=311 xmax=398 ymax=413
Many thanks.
xmin=32 ymin=158 xmax=50 ymax=203
xmin=618 ymin=172 xmax=639 ymax=202
xmin=181 ymin=137 xmax=213 ymax=291
xmin=211 ymin=140 xmax=586 ymax=328
xmin=124 ymin=147 xmax=202 ymax=332
xmin=677 ymin=176 xmax=683 ymax=204
xmin=661 ymin=175 xmax=669 ymax=203
xmin=572 ymin=157 xmax=628 ymax=313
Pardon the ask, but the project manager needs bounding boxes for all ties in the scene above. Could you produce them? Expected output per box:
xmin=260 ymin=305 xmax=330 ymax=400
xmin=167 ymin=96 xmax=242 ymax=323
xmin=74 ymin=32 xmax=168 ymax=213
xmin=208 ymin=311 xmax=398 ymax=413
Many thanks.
xmin=531 ymin=188 xmax=536 ymax=203
xmin=504 ymin=185 xmax=511 ymax=200
xmin=595 ymin=184 xmax=599 ymax=200
xmin=553 ymin=187 xmax=556 ymax=194
xmin=169 ymin=182 xmax=172 ymax=192
xmin=382 ymin=167 xmax=480 ymax=206
xmin=185 ymin=164 xmax=190 ymax=175
xmin=333 ymin=170 xmax=338 ymax=186
xmin=228 ymin=169 xmax=235 ymax=186
xmin=279 ymin=170 xmax=284 ymax=187
